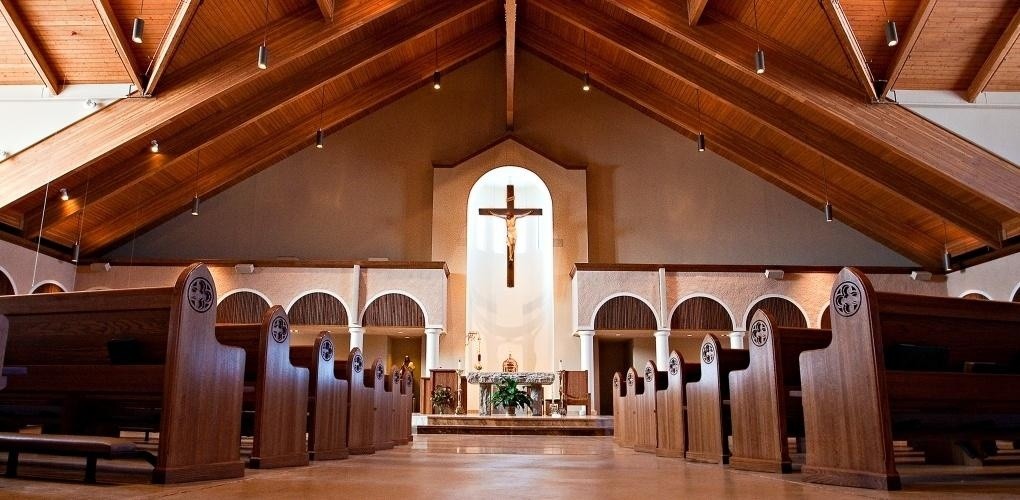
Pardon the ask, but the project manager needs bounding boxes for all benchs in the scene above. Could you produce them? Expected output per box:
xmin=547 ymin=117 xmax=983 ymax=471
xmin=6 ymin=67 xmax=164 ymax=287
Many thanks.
xmin=799 ymin=268 xmax=1020 ymax=490
xmin=613 ymin=307 xmax=997 ymax=474
xmin=1 ymin=263 xmax=246 ymax=486
xmin=213 ymin=305 xmax=413 ymax=470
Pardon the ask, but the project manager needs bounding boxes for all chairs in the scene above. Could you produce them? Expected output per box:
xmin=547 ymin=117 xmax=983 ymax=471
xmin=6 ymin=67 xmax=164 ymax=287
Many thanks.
xmin=558 ymin=370 xmax=592 ymax=415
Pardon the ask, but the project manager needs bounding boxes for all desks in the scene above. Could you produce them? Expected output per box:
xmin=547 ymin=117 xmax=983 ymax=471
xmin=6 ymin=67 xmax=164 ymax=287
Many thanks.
xmin=468 ymin=371 xmax=554 ymax=415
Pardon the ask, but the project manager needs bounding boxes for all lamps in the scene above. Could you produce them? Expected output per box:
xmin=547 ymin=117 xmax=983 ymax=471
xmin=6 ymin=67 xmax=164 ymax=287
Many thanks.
xmin=60 ymin=138 xmax=201 ymax=265
xmin=315 ymin=84 xmax=323 ymax=148
xmin=695 ymin=1 xmax=766 ymax=155
xmin=433 ymin=29 xmax=441 ymax=93
xmin=881 ymin=0 xmax=900 ymax=49
xmin=581 ymin=29 xmax=590 ymax=92
xmin=257 ymin=1 xmax=270 ymax=69
xmin=820 ymin=158 xmax=954 ymax=271
xmin=131 ymin=2 xmax=145 ymax=44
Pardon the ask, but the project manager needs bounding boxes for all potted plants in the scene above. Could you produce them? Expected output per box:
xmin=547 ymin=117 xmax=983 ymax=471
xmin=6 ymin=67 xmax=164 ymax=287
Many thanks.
xmin=427 ymin=382 xmax=455 ymax=414
xmin=489 ymin=376 xmax=536 ymax=416
